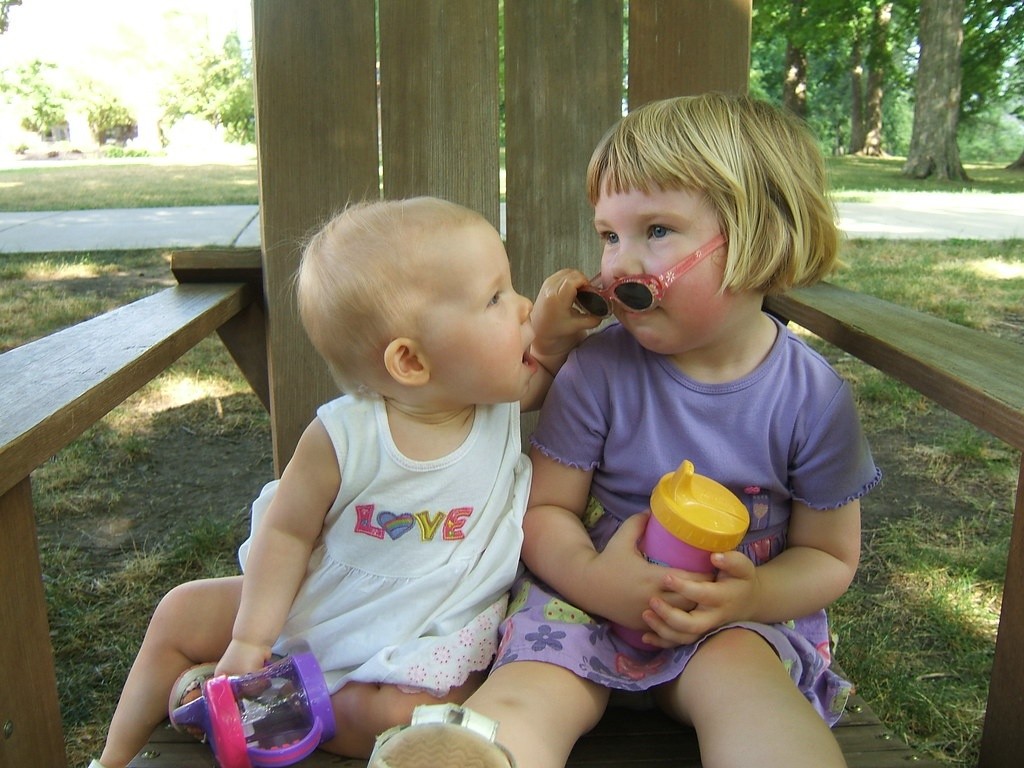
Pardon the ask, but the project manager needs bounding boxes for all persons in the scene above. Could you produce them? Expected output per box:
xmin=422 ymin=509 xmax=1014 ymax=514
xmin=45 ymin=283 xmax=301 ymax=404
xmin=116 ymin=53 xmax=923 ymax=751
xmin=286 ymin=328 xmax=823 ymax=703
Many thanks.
xmin=366 ymin=90 xmax=885 ymax=768
xmin=89 ymin=192 xmax=613 ymax=768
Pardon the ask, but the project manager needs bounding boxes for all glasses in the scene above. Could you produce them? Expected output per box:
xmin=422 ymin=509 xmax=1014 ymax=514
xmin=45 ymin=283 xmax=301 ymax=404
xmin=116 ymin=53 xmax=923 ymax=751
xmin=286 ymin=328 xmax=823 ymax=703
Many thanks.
xmin=574 ymin=230 xmax=728 ymax=320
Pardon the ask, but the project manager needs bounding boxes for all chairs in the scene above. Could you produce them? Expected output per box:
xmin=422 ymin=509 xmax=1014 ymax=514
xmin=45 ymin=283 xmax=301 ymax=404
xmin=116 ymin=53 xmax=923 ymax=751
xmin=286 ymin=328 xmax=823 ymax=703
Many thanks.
xmin=0 ymin=0 xmax=1024 ymax=768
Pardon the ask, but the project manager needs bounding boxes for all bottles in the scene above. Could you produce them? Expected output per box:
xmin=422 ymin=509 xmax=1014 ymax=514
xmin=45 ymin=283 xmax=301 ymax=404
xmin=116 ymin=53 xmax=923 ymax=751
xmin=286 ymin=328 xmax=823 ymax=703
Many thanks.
xmin=612 ymin=459 xmax=750 ymax=651
xmin=173 ymin=651 xmax=337 ymax=768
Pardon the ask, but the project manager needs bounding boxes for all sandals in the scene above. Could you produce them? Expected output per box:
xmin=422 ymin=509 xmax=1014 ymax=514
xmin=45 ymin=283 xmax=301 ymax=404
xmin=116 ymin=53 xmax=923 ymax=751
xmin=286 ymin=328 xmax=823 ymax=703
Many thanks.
xmin=367 ymin=701 xmax=516 ymax=768
xmin=168 ymin=660 xmax=292 ymax=744
